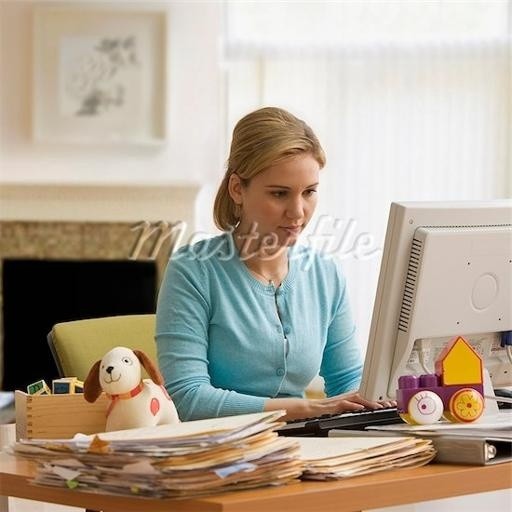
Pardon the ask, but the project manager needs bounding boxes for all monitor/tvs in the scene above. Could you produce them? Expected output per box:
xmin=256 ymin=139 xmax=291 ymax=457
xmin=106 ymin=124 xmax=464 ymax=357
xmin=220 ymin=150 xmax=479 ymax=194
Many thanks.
xmin=358 ymin=200 xmax=512 ymax=405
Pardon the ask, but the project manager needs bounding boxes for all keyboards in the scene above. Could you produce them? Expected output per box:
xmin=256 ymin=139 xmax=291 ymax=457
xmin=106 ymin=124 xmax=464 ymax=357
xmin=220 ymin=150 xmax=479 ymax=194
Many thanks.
xmin=274 ymin=408 xmax=405 ymax=436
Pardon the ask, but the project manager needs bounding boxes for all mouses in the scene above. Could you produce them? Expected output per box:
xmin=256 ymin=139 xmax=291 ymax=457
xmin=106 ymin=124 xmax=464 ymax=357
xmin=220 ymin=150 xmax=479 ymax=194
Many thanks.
xmin=494 ymin=389 xmax=512 ymax=409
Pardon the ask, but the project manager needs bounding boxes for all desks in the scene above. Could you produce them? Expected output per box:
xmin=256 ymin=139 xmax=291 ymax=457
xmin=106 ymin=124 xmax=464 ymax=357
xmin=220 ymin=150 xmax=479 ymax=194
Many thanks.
xmin=0 ymin=422 xmax=512 ymax=512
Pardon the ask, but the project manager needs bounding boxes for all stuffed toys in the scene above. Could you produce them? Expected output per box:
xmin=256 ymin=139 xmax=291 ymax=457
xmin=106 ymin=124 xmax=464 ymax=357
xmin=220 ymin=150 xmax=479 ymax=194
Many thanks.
xmin=85 ymin=346 xmax=180 ymax=432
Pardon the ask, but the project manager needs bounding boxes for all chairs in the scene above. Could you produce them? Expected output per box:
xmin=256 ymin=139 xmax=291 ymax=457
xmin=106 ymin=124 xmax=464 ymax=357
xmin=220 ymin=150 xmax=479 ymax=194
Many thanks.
xmin=47 ymin=313 xmax=164 ymax=387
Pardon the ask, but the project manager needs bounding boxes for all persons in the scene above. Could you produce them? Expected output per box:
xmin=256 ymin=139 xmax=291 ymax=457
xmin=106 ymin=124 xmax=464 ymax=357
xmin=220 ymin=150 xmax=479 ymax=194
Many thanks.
xmin=399 ymin=338 xmax=485 ymax=425
xmin=155 ymin=106 xmax=396 ymax=420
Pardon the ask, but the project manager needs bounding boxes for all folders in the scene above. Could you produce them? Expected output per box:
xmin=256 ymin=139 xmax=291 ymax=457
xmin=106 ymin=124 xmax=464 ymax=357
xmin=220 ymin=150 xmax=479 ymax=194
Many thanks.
xmin=328 ymin=428 xmax=512 ymax=465
